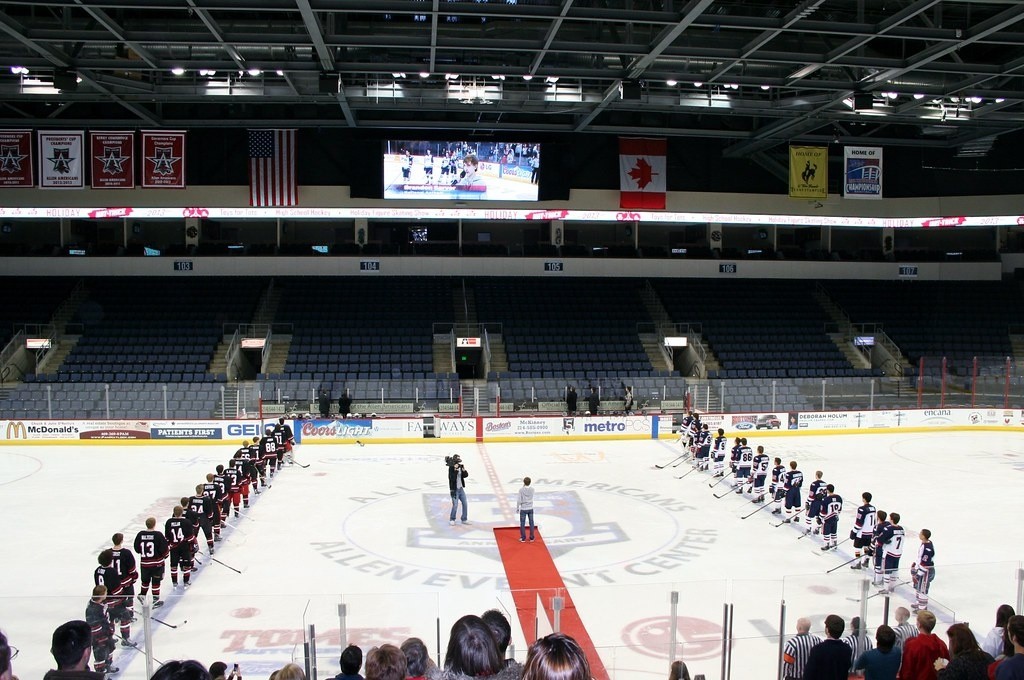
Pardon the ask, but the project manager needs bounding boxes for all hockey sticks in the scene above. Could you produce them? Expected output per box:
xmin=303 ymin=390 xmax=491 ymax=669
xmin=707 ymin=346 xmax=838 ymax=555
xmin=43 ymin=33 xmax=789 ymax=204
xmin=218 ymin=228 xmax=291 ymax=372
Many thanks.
xmin=701 ymin=465 xmax=734 ymax=489
xmin=673 ymin=457 xmax=711 ymax=479
xmin=796 ymin=523 xmax=823 ymax=539
xmin=810 ymin=536 xmax=851 ymax=556
xmin=283 ymin=453 xmax=310 ymax=468
xmin=126 ymin=607 xmax=188 ymax=629
xmin=230 ymin=506 xmax=260 ymax=522
xmin=211 ymin=531 xmax=247 ymax=546
xmin=220 ymin=519 xmax=250 ymax=535
xmin=193 ymin=549 xmax=241 ymax=574
xmin=655 ymin=451 xmax=693 ymax=469
xmin=769 ymin=508 xmax=808 ymax=527
xmin=713 ymin=480 xmax=749 ymax=499
xmin=824 ymin=552 xmax=868 ymax=574
xmin=113 ymin=632 xmax=165 ymax=667
xmin=254 ymin=465 xmax=272 ymax=489
xmin=735 ymin=492 xmax=776 ymax=519
xmin=848 ymin=580 xmax=914 ymax=603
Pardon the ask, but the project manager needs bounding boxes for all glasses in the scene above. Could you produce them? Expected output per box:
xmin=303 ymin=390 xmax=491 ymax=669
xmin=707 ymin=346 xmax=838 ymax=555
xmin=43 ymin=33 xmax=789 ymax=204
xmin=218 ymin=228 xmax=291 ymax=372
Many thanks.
xmin=8 ymin=646 xmax=20 ymax=660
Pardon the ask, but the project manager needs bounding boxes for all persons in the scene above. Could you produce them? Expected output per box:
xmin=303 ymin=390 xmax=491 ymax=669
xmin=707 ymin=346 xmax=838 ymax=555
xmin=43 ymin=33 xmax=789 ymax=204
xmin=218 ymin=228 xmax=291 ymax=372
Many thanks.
xmin=134 ymin=418 xmax=295 ymax=608
xmin=338 ymin=386 xmax=352 ymax=420
xmin=401 ymin=141 xmax=540 ymax=200
xmin=678 ymin=411 xmax=936 ymax=615
xmin=94 ymin=532 xmax=139 ymax=648
xmin=43 ymin=620 xmax=112 ymax=680
xmin=0 ymin=630 xmax=20 ymax=680
xmin=318 ymin=389 xmax=335 ymax=417
xmin=149 ymin=608 xmax=597 ymax=680
xmin=567 ymin=386 xmax=577 ymax=418
xmin=669 ymin=660 xmax=691 ymax=680
xmin=788 ymin=412 xmax=798 ymax=429
xmin=517 ymin=477 xmax=536 ymax=542
xmin=448 ymin=454 xmax=472 ymax=525
xmin=782 ymin=604 xmax=1024 ymax=680
xmin=86 ymin=584 xmax=119 ymax=675
xmin=622 ymin=385 xmax=633 ymax=415
xmin=584 ymin=386 xmax=600 ymax=416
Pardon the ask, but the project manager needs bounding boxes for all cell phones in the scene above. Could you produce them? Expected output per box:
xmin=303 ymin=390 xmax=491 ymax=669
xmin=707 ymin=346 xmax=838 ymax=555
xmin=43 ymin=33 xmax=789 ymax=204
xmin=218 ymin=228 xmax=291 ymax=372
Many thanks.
xmin=234 ymin=664 xmax=238 ymax=671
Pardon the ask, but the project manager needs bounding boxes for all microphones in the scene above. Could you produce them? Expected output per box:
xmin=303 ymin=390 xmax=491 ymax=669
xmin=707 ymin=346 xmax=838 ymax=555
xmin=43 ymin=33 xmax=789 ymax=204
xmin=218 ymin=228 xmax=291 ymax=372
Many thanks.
xmin=451 ymin=171 xmax=466 ymax=186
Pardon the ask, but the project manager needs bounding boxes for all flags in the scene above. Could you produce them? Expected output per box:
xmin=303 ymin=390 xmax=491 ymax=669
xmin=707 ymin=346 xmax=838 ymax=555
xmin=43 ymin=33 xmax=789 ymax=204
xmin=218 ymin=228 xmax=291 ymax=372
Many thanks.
xmin=618 ymin=134 xmax=669 ymax=212
xmin=249 ymin=129 xmax=298 ymax=207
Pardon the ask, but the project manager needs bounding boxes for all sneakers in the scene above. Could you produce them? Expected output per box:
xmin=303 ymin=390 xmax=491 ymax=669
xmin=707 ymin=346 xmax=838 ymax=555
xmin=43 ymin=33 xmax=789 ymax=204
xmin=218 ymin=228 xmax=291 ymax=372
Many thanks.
xmin=853 ymin=559 xmax=895 ymax=594
xmin=695 ymin=463 xmax=724 ymax=478
xmin=255 ymin=473 xmax=273 ymax=495
xmin=263 ymin=461 xmax=283 ymax=477
xmin=731 ymin=483 xmax=764 ymax=503
xmin=772 ymin=508 xmax=837 ymax=550
xmin=911 ymin=603 xmax=927 ymax=616
xmin=103 ymin=618 xmax=137 ymax=680
xmin=189 ymin=525 xmax=226 ymax=572
xmin=138 ymin=568 xmax=197 ymax=609
xmin=221 ymin=505 xmax=250 ymax=528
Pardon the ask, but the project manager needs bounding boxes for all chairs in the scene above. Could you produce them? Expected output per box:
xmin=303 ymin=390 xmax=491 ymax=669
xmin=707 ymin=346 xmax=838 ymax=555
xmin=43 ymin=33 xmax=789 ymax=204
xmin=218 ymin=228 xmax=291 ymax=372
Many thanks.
xmin=0 ymin=275 xmax=1024 ymax=420
xmin=0 ymin=242 xmax=1004 ymax=260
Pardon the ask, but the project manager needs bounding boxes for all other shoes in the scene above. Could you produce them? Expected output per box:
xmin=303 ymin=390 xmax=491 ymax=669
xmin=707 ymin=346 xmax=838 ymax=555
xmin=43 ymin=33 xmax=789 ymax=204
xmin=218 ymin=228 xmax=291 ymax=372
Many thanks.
xmin=449 ymin=520 xmax=455 ymax=525
xmin=518 ymin=538 xmax=526 ymax=542
xmin=529 ymin=537 xmax=535 ymax=541
xmin=462 ymin=520 xmax=472 ymax=524
